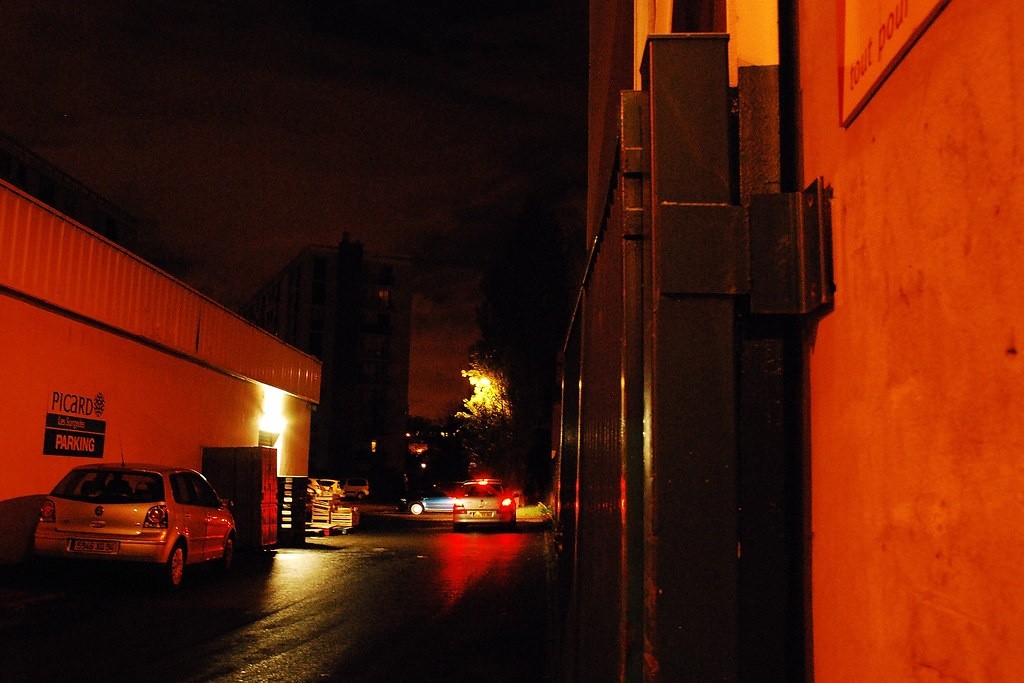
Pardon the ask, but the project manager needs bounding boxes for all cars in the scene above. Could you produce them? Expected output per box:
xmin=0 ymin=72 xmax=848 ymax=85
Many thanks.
xmin=33 ymin=463 xmax=238 ymax=595
xmin=337 ymin=478 xmax=370 ymax=500
xmin=453 ymin=480 xmax=520 ymax=530
xmin=406 ymin=480 xmax=462 ymax=514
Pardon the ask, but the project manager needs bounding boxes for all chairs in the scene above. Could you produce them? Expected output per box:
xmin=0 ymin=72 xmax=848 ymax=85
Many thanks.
xmin=107 ymin=479 xmax=133 ymax=496
